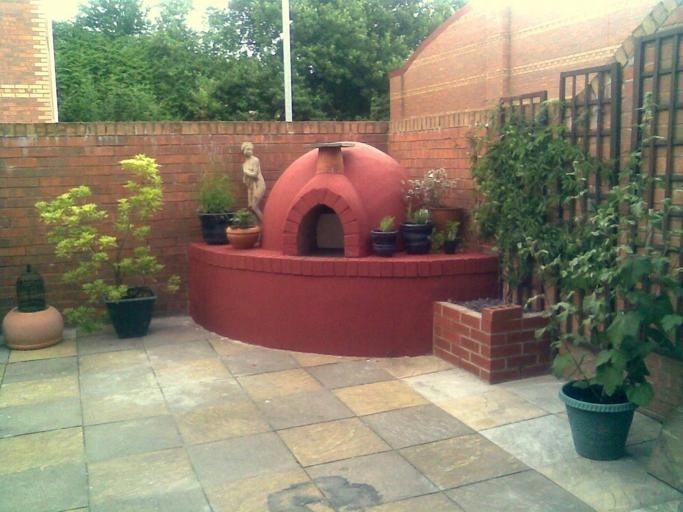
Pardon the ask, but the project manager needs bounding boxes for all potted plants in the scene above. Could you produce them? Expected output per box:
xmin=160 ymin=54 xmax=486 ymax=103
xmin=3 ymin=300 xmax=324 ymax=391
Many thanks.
xmin=518 ymin=92 xmax=683 ymax=461
xmin=197 ymin=176 xmax=237 ymax=244
xmin=34 ymin=153 xmax=181 ymax=339
xmin=370 ymin=168 xmax=463 ymax=257
xmin=226 ymin=208 xmax=260 ymax=249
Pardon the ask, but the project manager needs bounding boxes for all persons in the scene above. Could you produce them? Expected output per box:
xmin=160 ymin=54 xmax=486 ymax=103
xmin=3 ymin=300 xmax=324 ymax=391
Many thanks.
xmin=239 ymin=140 xmax=267 ymax=248
